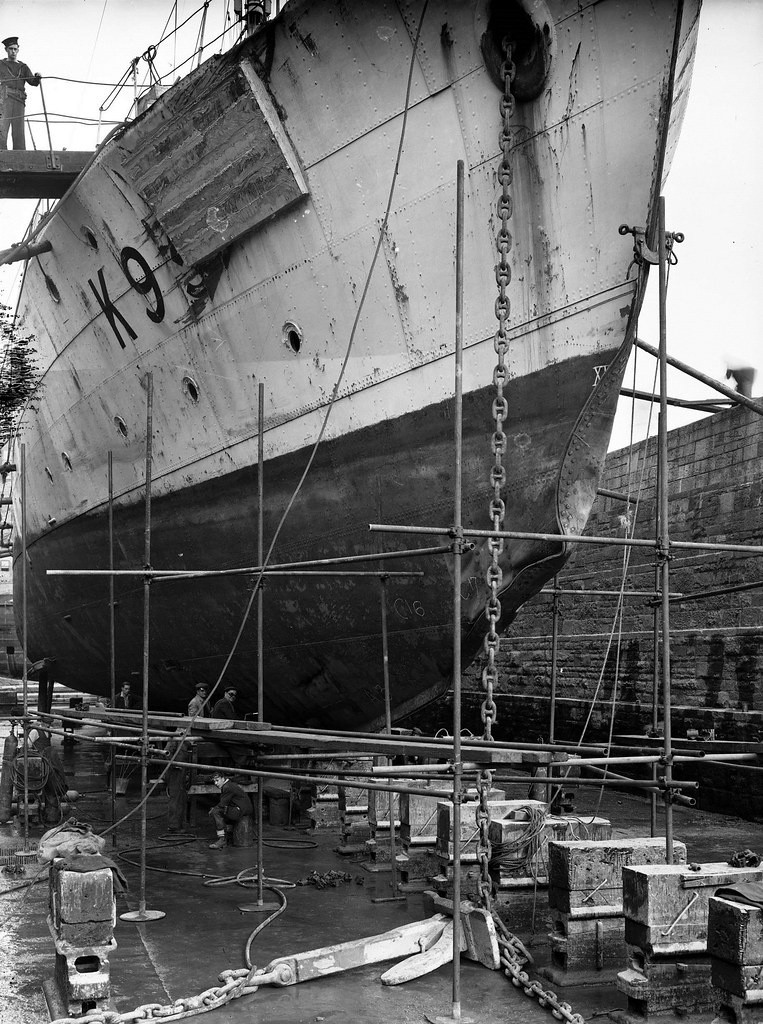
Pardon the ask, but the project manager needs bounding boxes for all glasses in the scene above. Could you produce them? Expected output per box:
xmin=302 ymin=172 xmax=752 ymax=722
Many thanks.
xmin=227 ymin=693 xmax=237 ymax=698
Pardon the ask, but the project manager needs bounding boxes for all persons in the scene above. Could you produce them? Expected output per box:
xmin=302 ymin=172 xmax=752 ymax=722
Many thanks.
xmin=188 ymin=680 xmax=239 ymax=720
xmin=0 ymin=37 xmax=42 ymax=150
xmin=164 ymin=727 xmax=191 ymax=832
xmin=106 ymin=682 xmax=137 ymax=710
xmin=27 ymin=716 xmax=55 ymax=754
xmin=209 ymin=771 xmax=251 ymax=851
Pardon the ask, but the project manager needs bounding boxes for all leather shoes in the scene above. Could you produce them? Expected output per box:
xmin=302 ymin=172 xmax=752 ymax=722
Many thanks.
xmin=209 ymin=837 xmax=228 ymax=849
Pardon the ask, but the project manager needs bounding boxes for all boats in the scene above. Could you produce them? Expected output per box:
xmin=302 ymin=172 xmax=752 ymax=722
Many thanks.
xmin=11 ymin=0 xmax=705 ymax=741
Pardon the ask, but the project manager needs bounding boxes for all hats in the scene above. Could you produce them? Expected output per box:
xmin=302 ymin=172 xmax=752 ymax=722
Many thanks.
xmin=224 ymin=686 xmax=237 ymax=692
xmin=195 ymin=682 xmax=209 ymax=688
